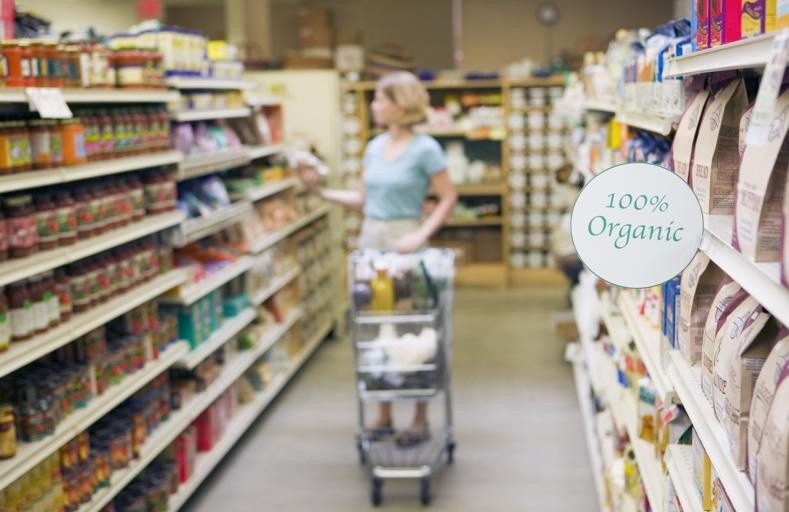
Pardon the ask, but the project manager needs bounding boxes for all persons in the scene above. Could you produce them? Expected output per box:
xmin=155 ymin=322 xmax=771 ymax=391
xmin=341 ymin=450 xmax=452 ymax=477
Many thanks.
xmin=297 ymin=70 xmax=457 ymax=449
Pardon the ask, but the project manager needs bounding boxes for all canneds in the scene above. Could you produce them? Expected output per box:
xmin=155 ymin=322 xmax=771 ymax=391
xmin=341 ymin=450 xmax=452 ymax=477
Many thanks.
xmin=0 ymin=39 xmax=177 ymax=512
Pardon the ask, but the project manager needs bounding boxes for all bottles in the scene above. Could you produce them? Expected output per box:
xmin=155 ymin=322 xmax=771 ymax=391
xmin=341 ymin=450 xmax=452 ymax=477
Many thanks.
xmin=371 ymin=268 xmax=393 ymax=309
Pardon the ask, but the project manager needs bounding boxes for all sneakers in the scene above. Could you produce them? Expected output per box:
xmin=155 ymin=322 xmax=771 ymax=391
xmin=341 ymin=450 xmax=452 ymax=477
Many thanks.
xmin=396 ymin=428 xmax=429 ymax=447
xmin=367 ymin=422 xmax=391 ymax=441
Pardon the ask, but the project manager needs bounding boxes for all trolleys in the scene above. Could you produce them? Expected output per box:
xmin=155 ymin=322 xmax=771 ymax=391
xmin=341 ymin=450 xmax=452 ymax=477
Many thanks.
xmin=348 ymin=248 xmax=454 ymax=506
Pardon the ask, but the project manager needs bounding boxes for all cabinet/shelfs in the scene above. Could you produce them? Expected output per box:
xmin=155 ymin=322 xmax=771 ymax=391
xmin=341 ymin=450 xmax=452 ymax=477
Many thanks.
xmin=340 ymin=73 xmax=571 ymax=290
xmin=0 ymin=77 xmax=332 ymax=503
xmin=570 ymin=28 xmax=789 ymax=511
